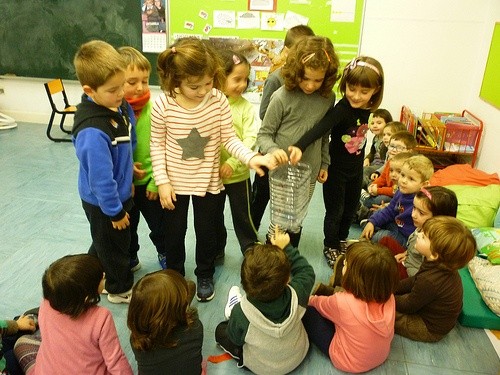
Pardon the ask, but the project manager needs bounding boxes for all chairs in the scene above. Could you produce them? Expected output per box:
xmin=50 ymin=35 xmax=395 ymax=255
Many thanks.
xmin=44 ymin=77 xmax=78 ymax=142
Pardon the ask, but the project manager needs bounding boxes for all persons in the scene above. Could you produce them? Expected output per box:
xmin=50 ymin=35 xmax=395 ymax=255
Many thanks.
xmin=377 ymin=186 xmax=458 ymax=281
xmin=310 ymin=217 xmax=477 ymax=342
xmin=355 ymin=130 xmax=417 ymax=218
xmin=361 ymin=152 xmax=415 ymax=231
xmin=303 ymin=241 xmax=396 ymax=374
xmin=363 ymin=109 xmax=393 ymax=167
xmin=349 ymin=154 xmax=433 ymax=245
xmin=255 ymin=36 xmax=340 ymax=249
xmin=127 ymin=269 xmax=206 ymax=375
xmin=288 ymin=55 xmax=384 ymax=269
xmin=150 ymin=36 xmax=276 ymax=302
xmin=213 ymin=50 xmax=258 ymax=264
xmin=361 ymin=121 xmax=408 ymax=188
xmin=251 ymin=24 xmax=315 ymax=243
xmin=116 ymin=46 xmax=169 ymax=272
xmin=214 ymin=225 xmax=315 ymax=375
xmin=15 ymin=253 xmax=133 ymax=375
xmin=0 ymin=308 xmax=39 ymax=375
xmin=72 ymin=40 xmax=137 ymax=304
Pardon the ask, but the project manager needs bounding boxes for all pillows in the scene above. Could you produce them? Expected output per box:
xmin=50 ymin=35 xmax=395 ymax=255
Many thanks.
xmin=471 ymin=226 xmax=500 ymax=264
xmin=429 ymin=164 xmax=500 ymax=186
xmin=468 ymin=256 xmax=500 ymax=317
xmin=444 ymin=183 xmax=500 ymax=228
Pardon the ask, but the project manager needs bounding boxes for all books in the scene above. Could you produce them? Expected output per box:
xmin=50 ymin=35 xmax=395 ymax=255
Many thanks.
xmin=442 ymin=116 xmax=475 ymax=154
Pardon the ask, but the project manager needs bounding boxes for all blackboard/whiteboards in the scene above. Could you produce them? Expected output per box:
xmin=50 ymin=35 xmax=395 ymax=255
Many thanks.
xmin=1 ymin=1 xmax=169 ymax=91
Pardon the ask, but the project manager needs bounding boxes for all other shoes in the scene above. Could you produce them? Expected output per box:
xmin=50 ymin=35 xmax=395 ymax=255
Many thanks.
xmin=158 ymin=253 xmax=167 ymax=270
xmin=197 ymin=276 xmax=215 ymax=302
xmin=361 ymin=188 xmax=371 ymax=201
xmin=340 ymin=240 xmax=348 ymax=255
xmin=312 ymin=282 xmax=334 ymax=296
xmin=323 ymin=246 xmax=341 ymax=269
xmin=225 ymin=285 xmax=242 ymax=318
xmin=108 ymin=289 xmax=132 ymax=304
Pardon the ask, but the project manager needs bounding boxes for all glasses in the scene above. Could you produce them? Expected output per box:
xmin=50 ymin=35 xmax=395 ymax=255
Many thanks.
xmin=387 ymin=144 xmax=407 ymax=152
